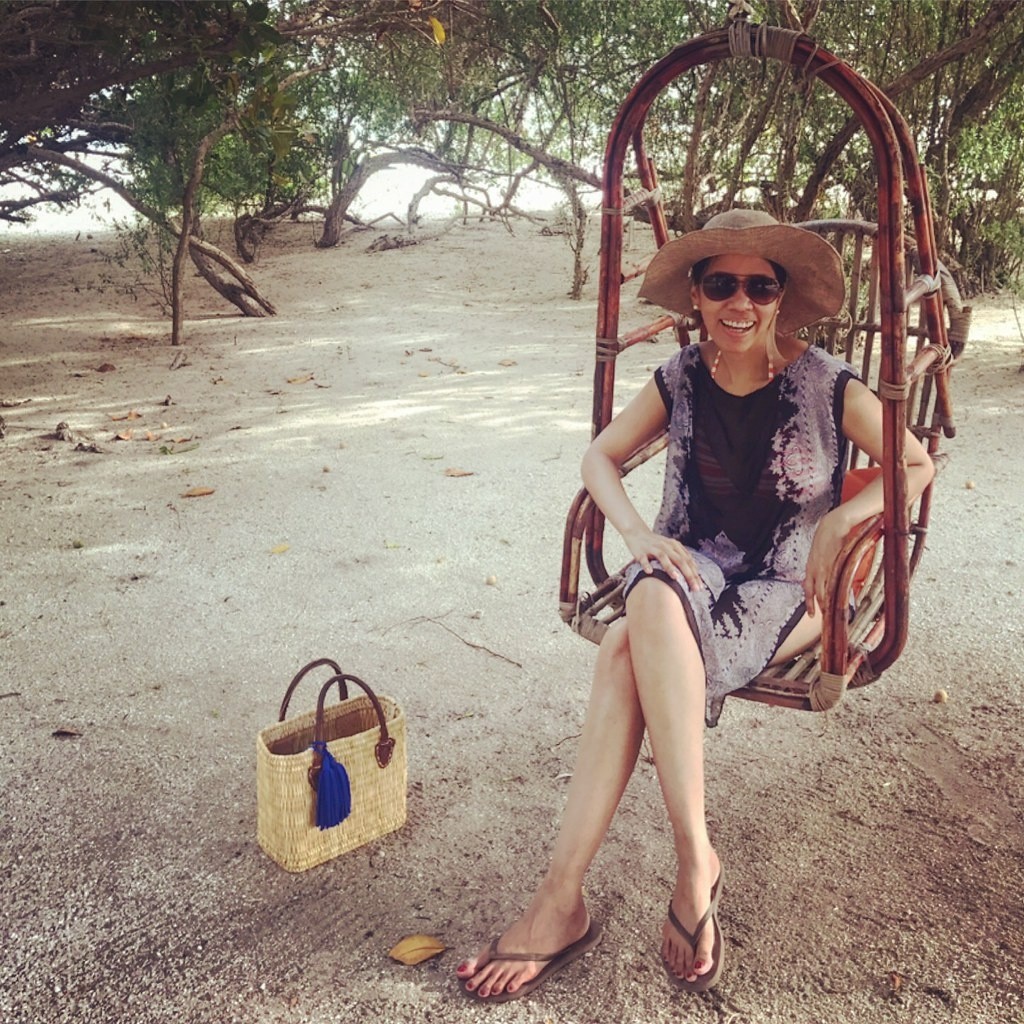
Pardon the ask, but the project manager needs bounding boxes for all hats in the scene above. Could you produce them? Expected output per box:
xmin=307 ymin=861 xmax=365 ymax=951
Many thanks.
xmin=636 ymin=208 xmax=847 ymax=335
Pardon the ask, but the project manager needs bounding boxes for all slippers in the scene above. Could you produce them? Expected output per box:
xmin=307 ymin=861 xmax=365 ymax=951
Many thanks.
xmin=456 ymin=915 xmax=603 ymax=1003
xmin=661 ymin=860 xmax=726 ymax=992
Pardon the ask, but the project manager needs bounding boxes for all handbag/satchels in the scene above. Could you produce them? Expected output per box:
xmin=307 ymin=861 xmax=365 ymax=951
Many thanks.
xmin=256 ymin=657 xmax=407 ymax=875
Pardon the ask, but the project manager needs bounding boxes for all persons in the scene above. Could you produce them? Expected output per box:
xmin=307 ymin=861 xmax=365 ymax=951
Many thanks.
xmin=452 ymin=202 xmax=937 ymax=1005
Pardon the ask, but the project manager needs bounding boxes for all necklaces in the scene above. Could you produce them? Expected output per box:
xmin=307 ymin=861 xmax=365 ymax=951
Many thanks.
xmin=709 ymin=348 xmax=774 ymax=390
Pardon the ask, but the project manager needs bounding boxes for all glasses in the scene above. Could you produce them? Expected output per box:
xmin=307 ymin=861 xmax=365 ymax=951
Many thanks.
xmin=693 ymin=270 xmax=784 ymax=307
